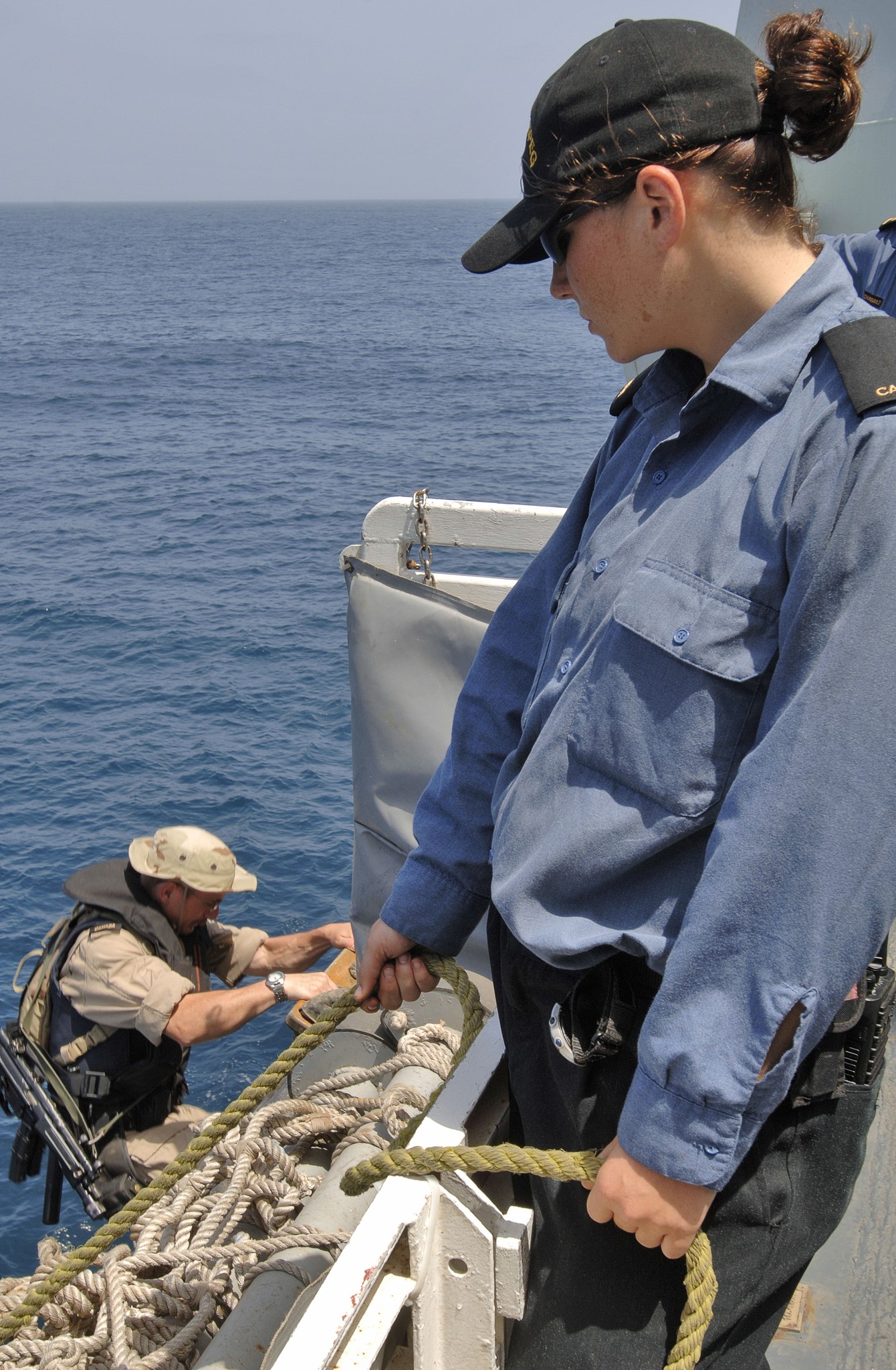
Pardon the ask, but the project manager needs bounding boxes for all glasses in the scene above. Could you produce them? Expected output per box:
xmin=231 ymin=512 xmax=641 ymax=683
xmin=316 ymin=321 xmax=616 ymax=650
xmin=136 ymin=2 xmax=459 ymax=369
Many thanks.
xmin=187 ymin=891 xmax=221 ymax=912
xmin=540 ymin=181 xmax=635 ymax=268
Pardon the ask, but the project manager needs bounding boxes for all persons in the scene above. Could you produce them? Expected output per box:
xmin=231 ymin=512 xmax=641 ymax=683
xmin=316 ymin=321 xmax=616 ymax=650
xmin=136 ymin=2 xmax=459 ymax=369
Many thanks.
xmin=45 ymin=826 xmax=356 ymax=1258
xmin=351 ymin=10 xmax=896 ymax=1370
xmin=811 ymin=218 xmax=896 ymax=318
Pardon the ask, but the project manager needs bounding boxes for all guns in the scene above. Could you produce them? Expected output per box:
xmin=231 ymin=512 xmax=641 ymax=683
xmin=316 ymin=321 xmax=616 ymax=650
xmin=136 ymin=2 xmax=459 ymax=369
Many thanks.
xmin=0 ymin=1021 xmax=110 ymax=1226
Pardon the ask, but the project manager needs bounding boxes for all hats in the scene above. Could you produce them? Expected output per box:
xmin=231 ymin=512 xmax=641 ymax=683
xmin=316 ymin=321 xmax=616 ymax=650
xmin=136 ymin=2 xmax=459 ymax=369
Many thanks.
xmin=128 ymin=825 xmax=259 ymax=893
xmin=461 ymin=18 xmax=765 ymax=274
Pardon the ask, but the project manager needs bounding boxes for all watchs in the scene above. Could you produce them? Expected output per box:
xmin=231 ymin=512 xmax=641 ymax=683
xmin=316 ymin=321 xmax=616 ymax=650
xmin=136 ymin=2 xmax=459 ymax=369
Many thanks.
xmin=265 ymin=971 xmax=287 ymax=1004
